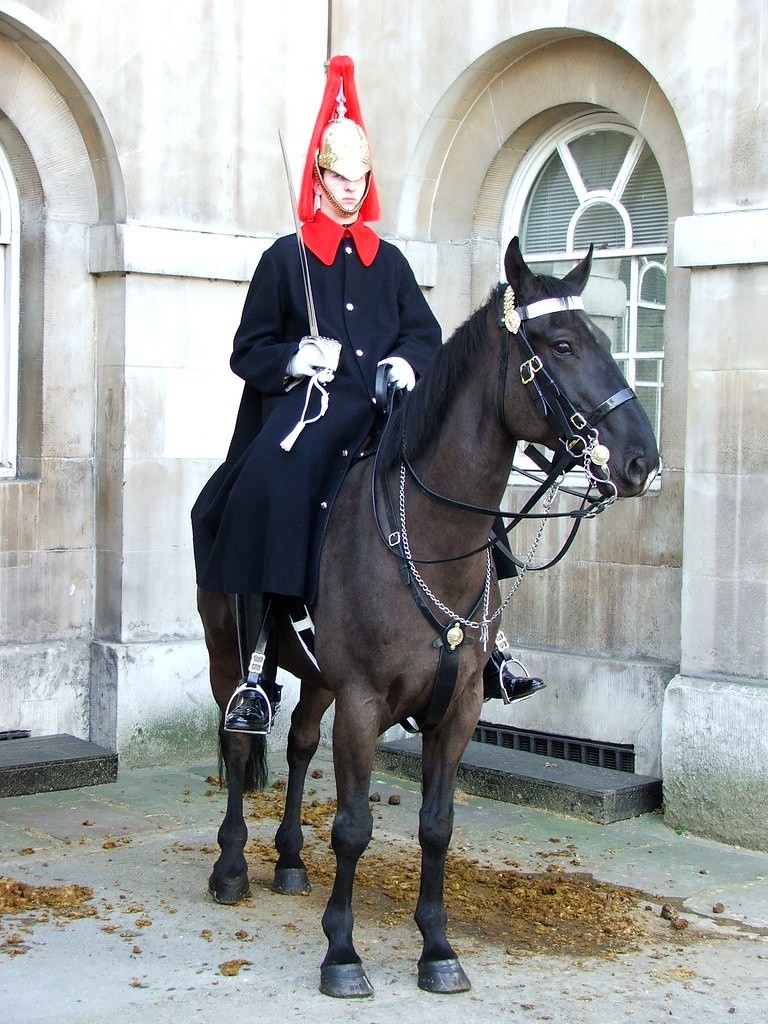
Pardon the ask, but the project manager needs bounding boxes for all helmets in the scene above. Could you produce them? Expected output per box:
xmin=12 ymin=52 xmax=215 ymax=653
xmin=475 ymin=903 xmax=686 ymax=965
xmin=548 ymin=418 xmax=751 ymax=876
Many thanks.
xmin=297 ymin=54 xmax=380 ymax=224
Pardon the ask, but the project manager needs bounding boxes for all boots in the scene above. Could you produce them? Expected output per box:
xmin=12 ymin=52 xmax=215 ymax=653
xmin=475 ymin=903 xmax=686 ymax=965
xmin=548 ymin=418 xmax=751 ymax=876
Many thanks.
xmin=482 ymin=651 xmax=547 ymax=701
xmin=226 ymin=590 xmax=278 ymax=731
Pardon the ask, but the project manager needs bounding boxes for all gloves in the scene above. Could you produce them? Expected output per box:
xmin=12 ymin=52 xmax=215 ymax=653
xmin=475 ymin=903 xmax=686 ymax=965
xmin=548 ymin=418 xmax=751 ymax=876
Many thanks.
xmin=377 ymin=357 xmax=416 ymax=392
xmin=286 ymin=340 xmax=327 ymax=379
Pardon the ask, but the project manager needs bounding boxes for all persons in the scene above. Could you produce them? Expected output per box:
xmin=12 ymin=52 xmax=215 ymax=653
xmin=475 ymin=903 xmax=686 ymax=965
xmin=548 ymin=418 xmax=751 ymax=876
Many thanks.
xmin=189 ymin=145 xmax=544 ymax=729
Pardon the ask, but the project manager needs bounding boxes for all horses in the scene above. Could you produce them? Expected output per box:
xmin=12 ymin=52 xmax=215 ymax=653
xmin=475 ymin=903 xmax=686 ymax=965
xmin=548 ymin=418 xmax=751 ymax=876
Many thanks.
xmin=187 ymin=235 xmax=664 ymax=997
